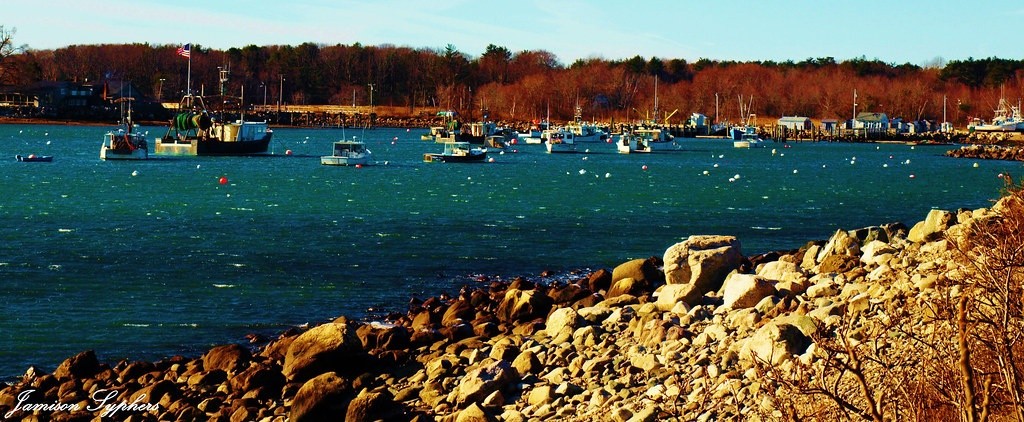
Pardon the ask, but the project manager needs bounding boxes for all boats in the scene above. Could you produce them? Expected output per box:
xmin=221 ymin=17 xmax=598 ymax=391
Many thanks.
xmin=155 ymin=93 xmax=275 ymax=156
xmin=524 ymin=128 xmax=553 ymax=144
xmin=561 ymin=124 xmax=610 ymax=141
xmin=966 ymin=99 xmax=1024 ymax=131
xmin=16 ymin=154 xmax=54 ymax=162
xmin=321 ymin=136 xmax=373 ymax=166
xmin=478 ymin=135 xmax=513 ymax=153
xmin=729 ymin=125 xmax=756 ymax=140
xmin=423 ymin=141 xmax=487 ymax=163
xmin=101 ymin=130 xmax=149 ymax=161
xmin=616 ymin=124 xmax=683 ymax=152
xmin=420 ymin=112 xmax=519 ymax=142
xmin=734 ymin=126 xmax=774 ymax=149
xmin=545 ymin=131 xmax=578 ymax=154
